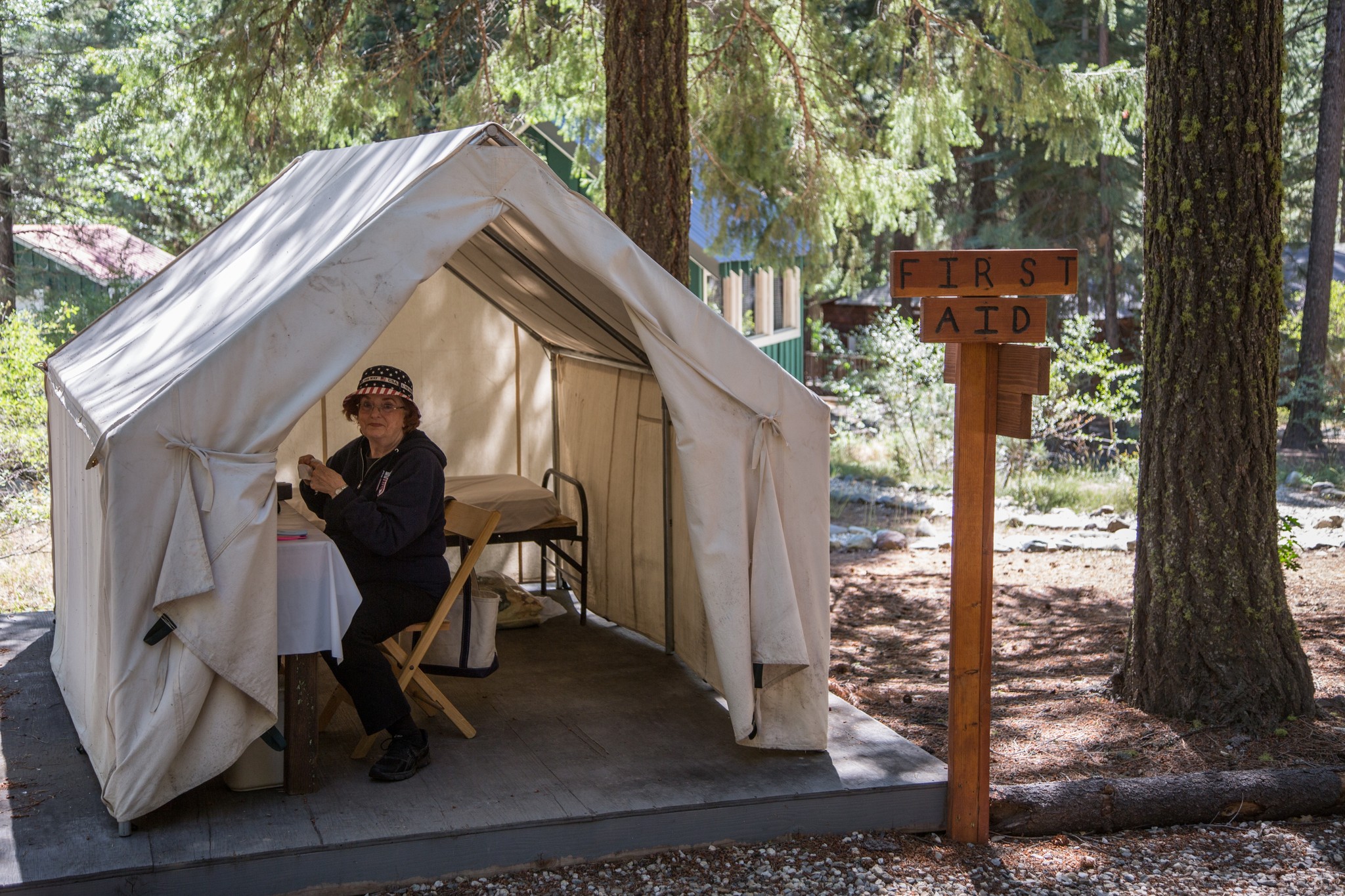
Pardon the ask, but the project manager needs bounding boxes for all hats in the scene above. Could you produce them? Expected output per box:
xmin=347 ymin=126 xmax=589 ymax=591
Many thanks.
xmin=342 ymin=364 xmax=422 ymax=420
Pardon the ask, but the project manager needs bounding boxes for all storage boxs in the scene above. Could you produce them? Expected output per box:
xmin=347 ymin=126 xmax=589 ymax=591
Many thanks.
xmin=219 ymin=667 xmax=289 ymax=793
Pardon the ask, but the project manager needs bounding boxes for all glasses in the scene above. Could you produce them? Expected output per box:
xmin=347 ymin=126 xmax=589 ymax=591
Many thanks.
xmin=356 ymin=402 xmax=407 ymax=413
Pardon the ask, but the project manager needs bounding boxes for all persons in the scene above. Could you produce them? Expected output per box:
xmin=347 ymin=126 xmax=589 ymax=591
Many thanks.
xmin=296 ymin=365 xmax=456 ymax=786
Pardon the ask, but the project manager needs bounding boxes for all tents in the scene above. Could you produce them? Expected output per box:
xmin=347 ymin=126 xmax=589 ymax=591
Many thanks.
xmin=43 ymin=120 xmax=843 ymax=835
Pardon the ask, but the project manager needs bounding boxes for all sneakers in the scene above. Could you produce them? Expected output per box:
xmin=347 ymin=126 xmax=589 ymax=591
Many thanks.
xmin=368 ymin=727 xmax=430 ymax=781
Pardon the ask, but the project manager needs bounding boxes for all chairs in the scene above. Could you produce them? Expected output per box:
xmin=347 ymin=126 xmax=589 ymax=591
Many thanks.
xmin=317 ymin=491 xmax=502 ymax=764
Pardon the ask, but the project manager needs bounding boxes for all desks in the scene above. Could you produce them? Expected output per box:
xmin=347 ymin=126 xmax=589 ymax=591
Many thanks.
xmin=256 ymin=495 xmax=368 ymax=794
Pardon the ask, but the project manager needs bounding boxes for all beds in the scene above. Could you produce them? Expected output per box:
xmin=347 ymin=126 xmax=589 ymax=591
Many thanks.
xmin=437 ymin=466 xmax=592 ymax=629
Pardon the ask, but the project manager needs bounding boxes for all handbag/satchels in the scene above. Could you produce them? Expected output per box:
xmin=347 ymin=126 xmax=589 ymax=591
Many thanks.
xmin=396 ymin=495 xmax=501 ymax=679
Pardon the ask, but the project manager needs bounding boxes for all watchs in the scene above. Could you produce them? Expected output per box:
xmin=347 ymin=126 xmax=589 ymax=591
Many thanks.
xmin=331 ymin=484 xmax=348 ymax=499
